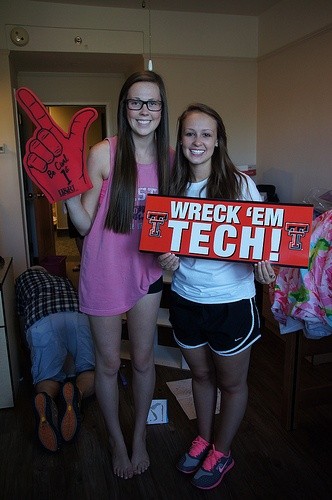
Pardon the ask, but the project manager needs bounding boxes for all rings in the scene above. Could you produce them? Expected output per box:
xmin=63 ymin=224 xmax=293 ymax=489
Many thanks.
xmin=269 ymin=274 xmax=276 ymax=280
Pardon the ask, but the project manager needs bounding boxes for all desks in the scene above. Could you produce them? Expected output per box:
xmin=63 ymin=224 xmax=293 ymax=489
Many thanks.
xmin=257 ymin=266 xmax=332 ymax=431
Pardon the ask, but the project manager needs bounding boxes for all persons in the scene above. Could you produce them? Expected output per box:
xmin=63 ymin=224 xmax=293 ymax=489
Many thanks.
xmin=63 ymin=70 xmax=177 ymax=481
xmin=155 ymin=101 xmax=277 ymax=490
xmin=14 ymin=266 xmax=96 ymax=453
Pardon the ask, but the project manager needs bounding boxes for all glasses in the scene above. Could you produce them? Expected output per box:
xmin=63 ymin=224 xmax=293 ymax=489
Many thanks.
xmin=126 ymin=99 xmax=163 ymax=112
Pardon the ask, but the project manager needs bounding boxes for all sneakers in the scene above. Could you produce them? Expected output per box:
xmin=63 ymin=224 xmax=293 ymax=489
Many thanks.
xmin=176 ymin=432 xmax=214 ymax=474
xmin=58 ymin=381 xmax=81 ymax=445
xmin=189 ymin=444 xmax=235 ymax=489
xmin=32 ymin=392 xmax=62 ymax=453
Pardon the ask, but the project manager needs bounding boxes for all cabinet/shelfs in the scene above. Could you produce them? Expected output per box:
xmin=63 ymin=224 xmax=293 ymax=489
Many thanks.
xmin=0 ymin=258 xmax=24 ymax=410
